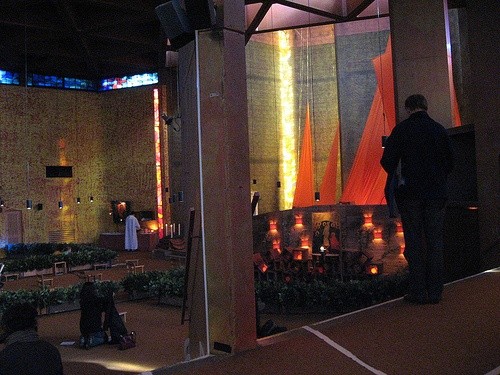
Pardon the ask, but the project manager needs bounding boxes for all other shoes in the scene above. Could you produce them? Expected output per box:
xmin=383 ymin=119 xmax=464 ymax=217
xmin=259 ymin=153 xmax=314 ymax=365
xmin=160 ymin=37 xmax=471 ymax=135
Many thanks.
xmin=427 ymin=293 xmax=442 ymax=304
xmin=79 ymin=335 xmax=85 ymax=348
xmin=86 ymin=335 xmax=94 ymax=350
xmin=404 ymin=293 xmax=425 ymax=304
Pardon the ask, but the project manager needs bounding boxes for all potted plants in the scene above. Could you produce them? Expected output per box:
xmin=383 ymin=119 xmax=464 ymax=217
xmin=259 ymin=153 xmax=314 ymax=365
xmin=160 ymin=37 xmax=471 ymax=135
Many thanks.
xmin=0 ymin=243 xmax=186 ymax=319
xmin=256 ymin=271 xmax=418 ymax=313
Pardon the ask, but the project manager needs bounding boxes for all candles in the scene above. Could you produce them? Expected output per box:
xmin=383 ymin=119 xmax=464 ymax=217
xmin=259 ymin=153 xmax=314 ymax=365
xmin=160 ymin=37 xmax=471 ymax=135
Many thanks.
xmin=173 ymin=223 xmax=175 ymax=232
xmin=166 ymin=224 xmax=167 ymax=236
xmin=170 ymin=224 xmax=173 ymax=238
xmin=178 ymin=224 xmax=180 ymax=235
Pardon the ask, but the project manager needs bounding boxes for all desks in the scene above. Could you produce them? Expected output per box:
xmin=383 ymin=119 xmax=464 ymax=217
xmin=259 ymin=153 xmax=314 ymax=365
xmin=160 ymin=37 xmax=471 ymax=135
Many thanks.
xmin=53 ymin=262 xmax=66 ymax=276
xmin=100 ymin=233 xmax=156 ymax=251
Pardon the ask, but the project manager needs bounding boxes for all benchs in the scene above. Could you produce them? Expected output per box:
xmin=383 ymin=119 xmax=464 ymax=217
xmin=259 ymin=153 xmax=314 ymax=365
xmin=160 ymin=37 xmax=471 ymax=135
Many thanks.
xmin=94 ymin=264 xmax=108 ymax=271
xmin=131 ymin=265 xmax=144 ymax=273
xmin=87 ymin=272 xmax=102 ymax=281
xmin=2 ymin=273 xmax=20 ymax=282
xmin=126 ymin=260 xmax=138 ymax=269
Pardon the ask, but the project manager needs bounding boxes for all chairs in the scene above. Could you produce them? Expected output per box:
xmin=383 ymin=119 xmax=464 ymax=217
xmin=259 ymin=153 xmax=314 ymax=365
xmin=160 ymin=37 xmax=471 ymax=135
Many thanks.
xmin=36 ymin=273 xmax=53 ymax=288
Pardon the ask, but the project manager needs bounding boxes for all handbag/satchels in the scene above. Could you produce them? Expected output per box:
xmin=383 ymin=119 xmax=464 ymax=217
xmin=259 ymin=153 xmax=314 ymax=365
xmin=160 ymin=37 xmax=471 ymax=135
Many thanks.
xmin=119 ymin=330 xmax=137 ymax=350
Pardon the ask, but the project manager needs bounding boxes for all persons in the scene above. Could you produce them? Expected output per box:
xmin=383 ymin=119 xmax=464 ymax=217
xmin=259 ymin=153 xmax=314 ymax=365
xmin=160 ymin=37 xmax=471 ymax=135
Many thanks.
xmin=380 ymin=95 xmax=455 ymax=306
xmin=124 ymin=212 xmax=141 ymax=252
xmin=76 ymin=282 xmax=128 ymax=349
xmin=0 ymin=304 xmax=64 ymax=375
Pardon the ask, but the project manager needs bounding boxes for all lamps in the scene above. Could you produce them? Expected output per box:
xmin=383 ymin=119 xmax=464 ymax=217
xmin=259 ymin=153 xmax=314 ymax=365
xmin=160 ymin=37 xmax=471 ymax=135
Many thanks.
xmin=77 ymin=197 xmax=81 ymax=204
xmin=277 ymin=181 xmax=280 ymax=187
xmin=315 ymin=192 xmax=320 ymax=201
xmin=253 ymin=179 xmax=256 ymax=184
xmin=90 ymin=197 xmax=93 ymax=202
xmin=161 ymin=113 xmax=181 ymax=124
xmin=58 ymin=201 xmax=63 ymax=209
xmin=382 ymin=136 xmax=389 ymax=148
xmin=27 ymin=199 xmax=32 ymax=210
